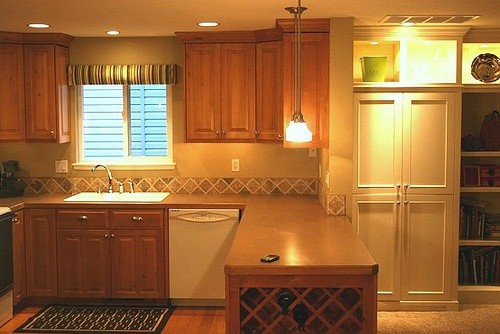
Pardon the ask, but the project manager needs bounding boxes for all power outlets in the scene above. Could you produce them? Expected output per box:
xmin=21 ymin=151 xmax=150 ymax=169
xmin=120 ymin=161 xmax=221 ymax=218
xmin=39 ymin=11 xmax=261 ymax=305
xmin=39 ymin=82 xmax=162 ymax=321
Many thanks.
xmin=232 ymin=159 xmax=239 ymax=171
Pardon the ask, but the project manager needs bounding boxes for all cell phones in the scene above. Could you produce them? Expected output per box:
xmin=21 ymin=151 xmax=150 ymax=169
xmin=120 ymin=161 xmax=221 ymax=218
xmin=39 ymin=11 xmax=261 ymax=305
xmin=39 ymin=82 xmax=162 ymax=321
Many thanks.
xmin=261 ymin=255 xmax=279 ymax=262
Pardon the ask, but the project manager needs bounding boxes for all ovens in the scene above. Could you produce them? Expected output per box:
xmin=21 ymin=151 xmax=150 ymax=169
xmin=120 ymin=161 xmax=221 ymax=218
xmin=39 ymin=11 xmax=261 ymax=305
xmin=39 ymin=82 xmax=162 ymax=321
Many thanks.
xmin=0 ymin=211 xmax=17 ymax=297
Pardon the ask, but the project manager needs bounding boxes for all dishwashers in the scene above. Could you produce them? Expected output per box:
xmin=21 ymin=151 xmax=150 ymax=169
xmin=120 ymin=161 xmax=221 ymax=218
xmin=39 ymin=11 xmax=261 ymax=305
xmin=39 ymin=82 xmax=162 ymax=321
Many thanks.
xmin=169 ymin=209 xmax=239 ymax=306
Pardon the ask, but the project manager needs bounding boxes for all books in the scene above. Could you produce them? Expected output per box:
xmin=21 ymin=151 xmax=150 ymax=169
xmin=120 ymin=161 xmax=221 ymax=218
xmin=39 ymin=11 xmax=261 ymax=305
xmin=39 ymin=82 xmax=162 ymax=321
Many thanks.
xmin=458 ymin=197 xmax=500 ymax=287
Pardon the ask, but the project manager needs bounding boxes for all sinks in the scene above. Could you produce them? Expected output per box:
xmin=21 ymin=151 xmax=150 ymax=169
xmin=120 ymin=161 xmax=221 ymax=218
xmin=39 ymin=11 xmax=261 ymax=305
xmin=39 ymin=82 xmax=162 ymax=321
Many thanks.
xmin=119 ymin=192 xmax=163 ymax=201
xmin=70 ymin=193 xmax=116 ymax=200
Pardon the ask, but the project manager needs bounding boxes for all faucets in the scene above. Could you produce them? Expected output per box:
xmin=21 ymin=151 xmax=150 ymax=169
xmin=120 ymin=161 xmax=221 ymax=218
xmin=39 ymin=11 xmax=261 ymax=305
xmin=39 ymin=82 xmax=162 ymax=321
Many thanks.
xmin=91 ymin=163 xmax=114 ymax=193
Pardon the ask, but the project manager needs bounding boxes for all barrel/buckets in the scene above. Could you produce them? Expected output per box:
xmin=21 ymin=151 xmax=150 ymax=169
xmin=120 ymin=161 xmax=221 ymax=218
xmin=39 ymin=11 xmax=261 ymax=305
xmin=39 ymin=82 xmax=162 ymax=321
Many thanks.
xmin=360 ymin=57 xmax=388 ymax=82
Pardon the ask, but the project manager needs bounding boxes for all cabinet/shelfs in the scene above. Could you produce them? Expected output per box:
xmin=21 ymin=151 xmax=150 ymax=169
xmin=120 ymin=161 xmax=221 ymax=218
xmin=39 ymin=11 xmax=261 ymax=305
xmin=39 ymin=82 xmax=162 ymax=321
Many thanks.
xmin=254 ymin=28 xmax=283 ymax=145
xmin=11 ymin=209 xmax=27 ymax=314
xmin=276 ymin=18 xmax=331 ymax=149
xmin=24 ymin=208 xmax=57 ymax=305
xmin=174 ymin=31 xmax=255 ymax=143
xmin=23 ymin=33 xmax=75 ymax=144
xmin=56 ymin=209 xmax=170 ymax=305
xmin=352 ymin=22 xmax=472 ymax=311
xmin=0 ymin=31 xmax=27 ymax=144
xmin=457 ymin=28 xmax=500 ymax=305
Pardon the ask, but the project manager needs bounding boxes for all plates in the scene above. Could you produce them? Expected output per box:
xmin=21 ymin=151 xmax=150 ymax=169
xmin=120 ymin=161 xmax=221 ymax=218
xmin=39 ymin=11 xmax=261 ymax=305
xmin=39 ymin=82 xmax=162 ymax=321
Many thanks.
xmin=471 ymin=53 xmax=500 ymax=82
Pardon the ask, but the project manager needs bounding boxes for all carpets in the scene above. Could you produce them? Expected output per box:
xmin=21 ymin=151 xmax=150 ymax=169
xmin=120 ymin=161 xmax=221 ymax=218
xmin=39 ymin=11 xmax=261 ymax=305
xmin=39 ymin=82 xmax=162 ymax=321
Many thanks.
xmin=12 ymin=304 xmax=177 ymax=334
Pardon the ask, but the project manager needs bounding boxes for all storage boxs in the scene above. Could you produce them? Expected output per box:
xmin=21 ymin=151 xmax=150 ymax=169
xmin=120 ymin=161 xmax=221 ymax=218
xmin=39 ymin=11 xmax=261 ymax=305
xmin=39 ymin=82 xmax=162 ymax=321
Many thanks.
xmin=480 ymin=165 xmax=500 ymax=187
xmin=359 ymin=56 xmax=388 ymax=82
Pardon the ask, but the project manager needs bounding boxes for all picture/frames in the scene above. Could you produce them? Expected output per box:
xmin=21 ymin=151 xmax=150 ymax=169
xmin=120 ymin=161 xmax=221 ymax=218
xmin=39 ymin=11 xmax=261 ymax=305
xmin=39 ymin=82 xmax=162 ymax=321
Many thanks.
xmin=463 ymin=166 xmax=481 ymax=187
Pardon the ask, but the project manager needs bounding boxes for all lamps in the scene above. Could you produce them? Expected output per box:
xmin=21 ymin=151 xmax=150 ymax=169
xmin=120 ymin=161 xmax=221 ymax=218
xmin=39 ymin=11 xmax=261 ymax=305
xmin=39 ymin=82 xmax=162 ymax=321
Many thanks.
xmin=284 ymin=0 xmax=313 ymax=143
